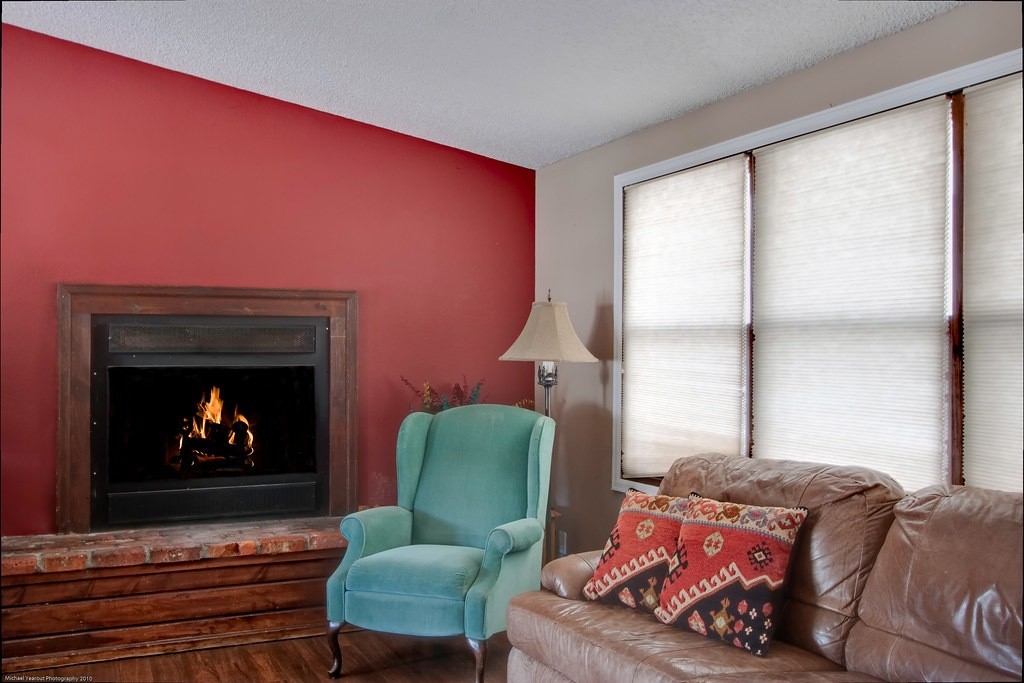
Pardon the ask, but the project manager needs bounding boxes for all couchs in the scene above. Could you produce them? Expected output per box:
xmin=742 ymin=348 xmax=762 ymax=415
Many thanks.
xmin=507 ymin=453 xmax=1023 ymax=683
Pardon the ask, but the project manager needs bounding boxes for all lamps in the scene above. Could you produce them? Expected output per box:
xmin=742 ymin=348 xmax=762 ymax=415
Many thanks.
xmin=498 ymin=288 xmax=599 ymax=561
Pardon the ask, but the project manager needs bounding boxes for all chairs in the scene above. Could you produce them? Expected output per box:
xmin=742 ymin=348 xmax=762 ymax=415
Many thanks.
xmin=326 ymin=405 xmax=557 ymax=683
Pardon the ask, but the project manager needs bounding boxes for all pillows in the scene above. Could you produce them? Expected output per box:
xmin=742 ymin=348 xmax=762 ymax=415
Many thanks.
xmin=654 ymin=492 xmax=808 ymax=658
xmin=582 ymin=488 xmax=688 ymax=613
